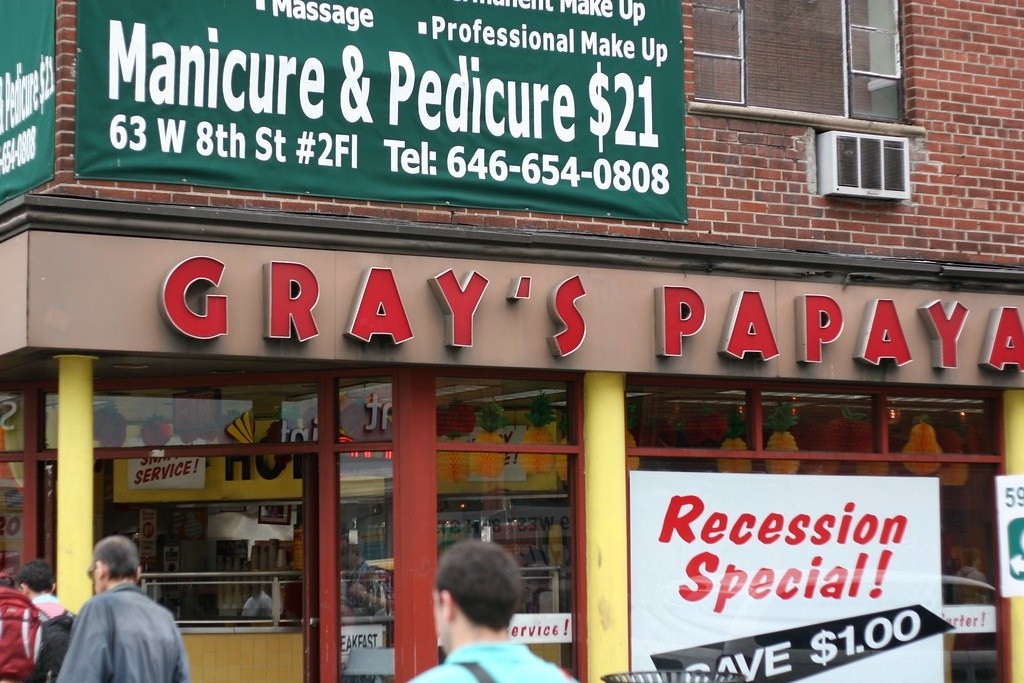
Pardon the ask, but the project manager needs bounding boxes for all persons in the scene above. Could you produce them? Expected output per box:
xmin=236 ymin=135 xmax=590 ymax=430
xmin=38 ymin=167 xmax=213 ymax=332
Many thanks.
xmin=404 ymin=540 xmax=577 ymax=682
xmin=0 ymin=548 xmax=44 ymax=683
xmin=16 ymin=560 xmax=77 ymax=682
xmin=521 ymin=549 xmax=560 ymax=613
xmin=953 ymin=549 xmax=991 ymax=604
xmin=341 ymin=534 xmax=387 ymax=616
xmin=56 ymin=536 xmax=190 ymax=683
xmin=240 ymin=570 xmax=273 ymax=618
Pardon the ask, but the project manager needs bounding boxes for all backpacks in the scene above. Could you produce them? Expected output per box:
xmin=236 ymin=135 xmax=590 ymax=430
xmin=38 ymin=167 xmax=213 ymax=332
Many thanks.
xmin=0 ymin=574 xmax=41 ymax=683
xmin=24 ymin=607 xmax=74 ymax=683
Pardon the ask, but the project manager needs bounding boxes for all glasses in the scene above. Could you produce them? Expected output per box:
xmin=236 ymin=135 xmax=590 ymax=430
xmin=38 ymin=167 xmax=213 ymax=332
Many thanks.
xmin=89 ymin=566 xmax=96 ymax=577
